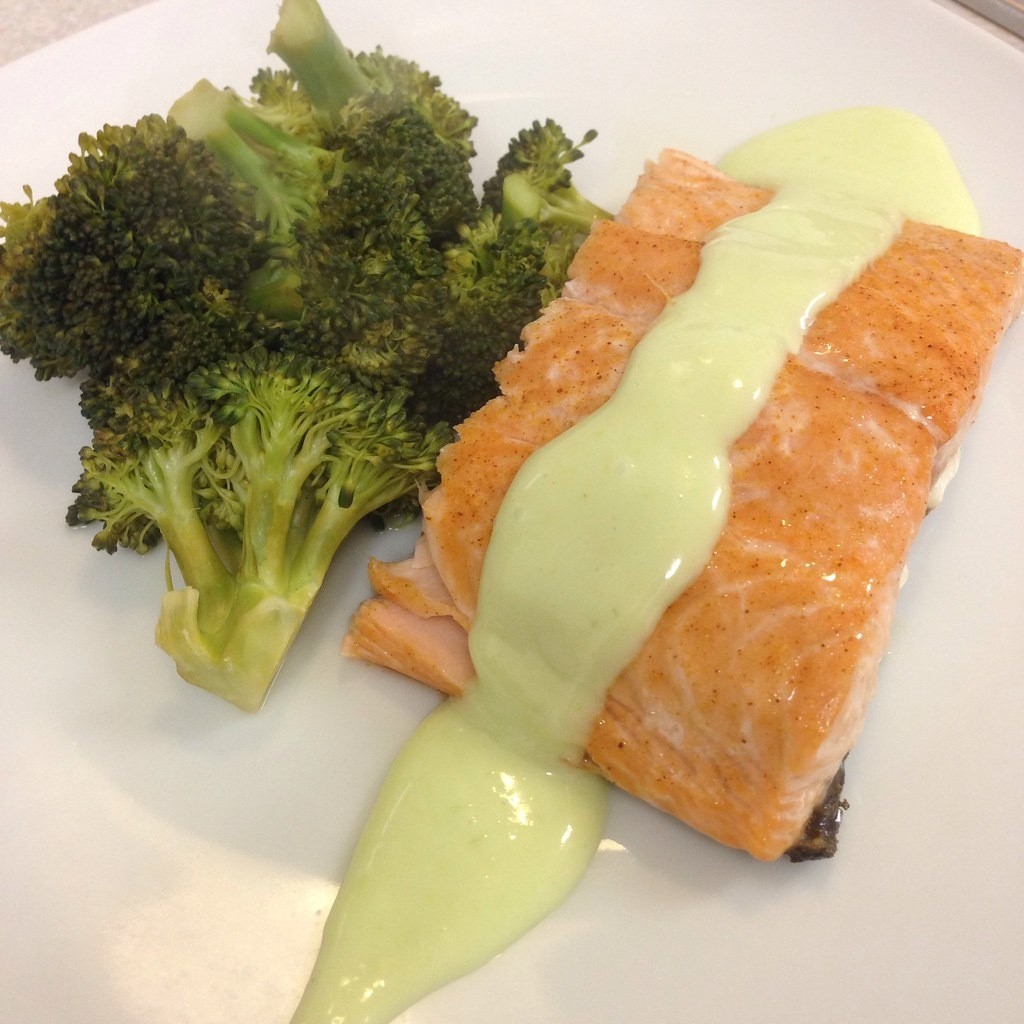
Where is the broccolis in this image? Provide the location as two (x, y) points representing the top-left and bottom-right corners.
(0, 0), (621, 714)
